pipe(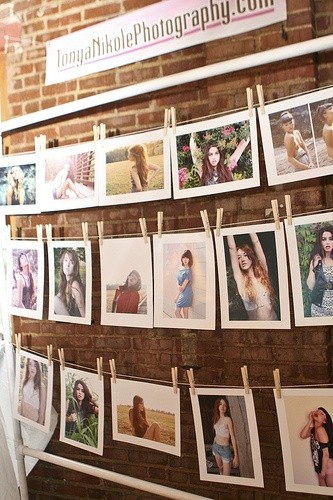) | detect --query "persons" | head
[16,226,333,317]
[5,103,333,206]
[20,358,333,487]
